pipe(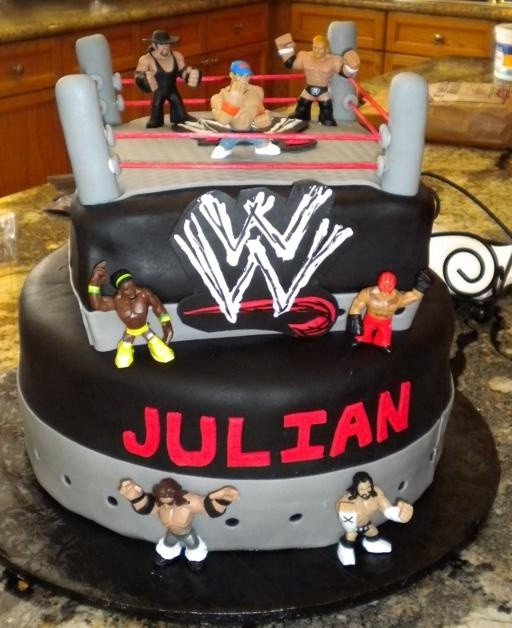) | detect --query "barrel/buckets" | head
[494,24,512,80]
